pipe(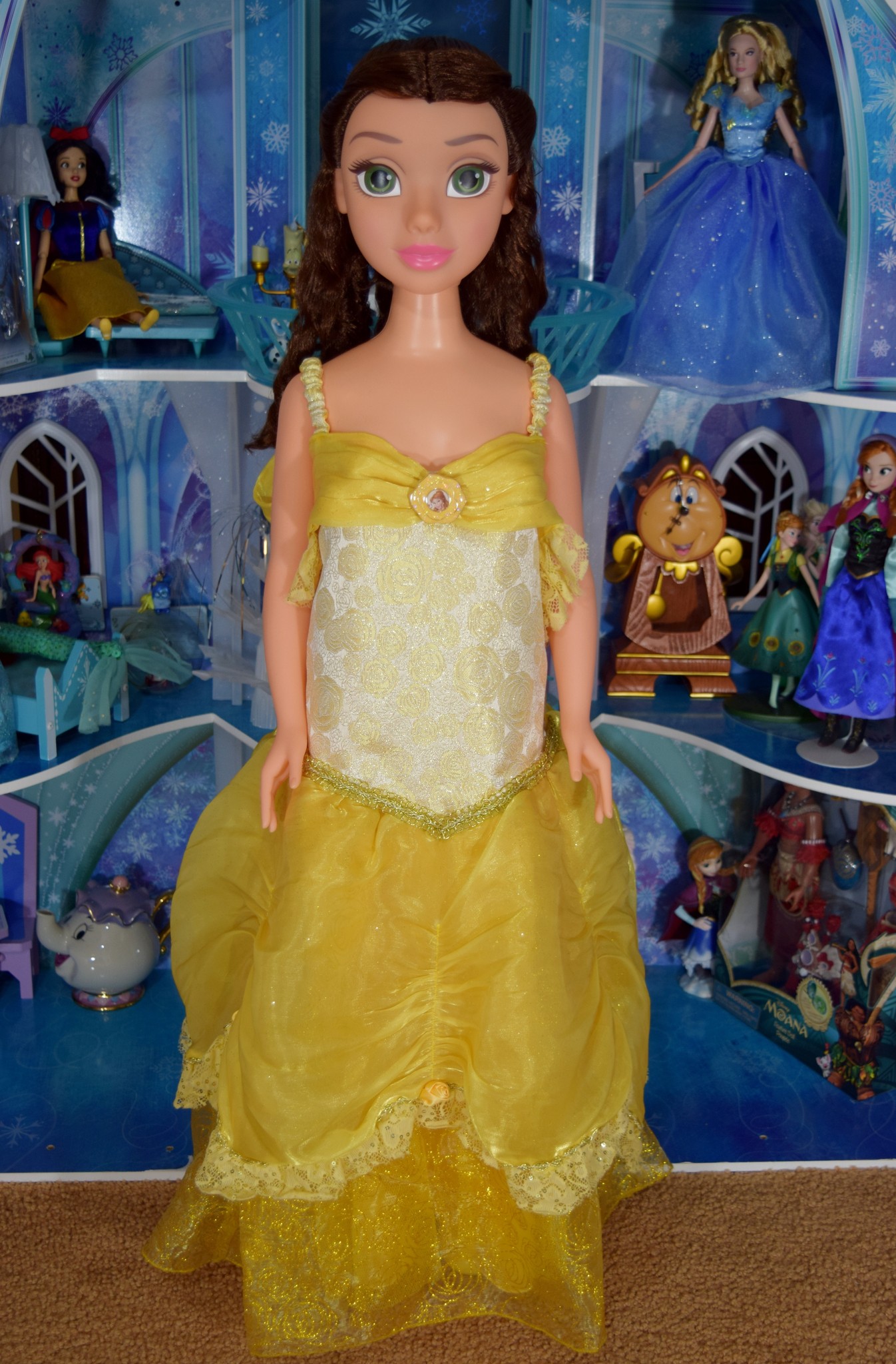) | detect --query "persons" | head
[3,427,895,1110]
[139,33,673,1364]
[31,123,159,348]
[585,14,849,400]
